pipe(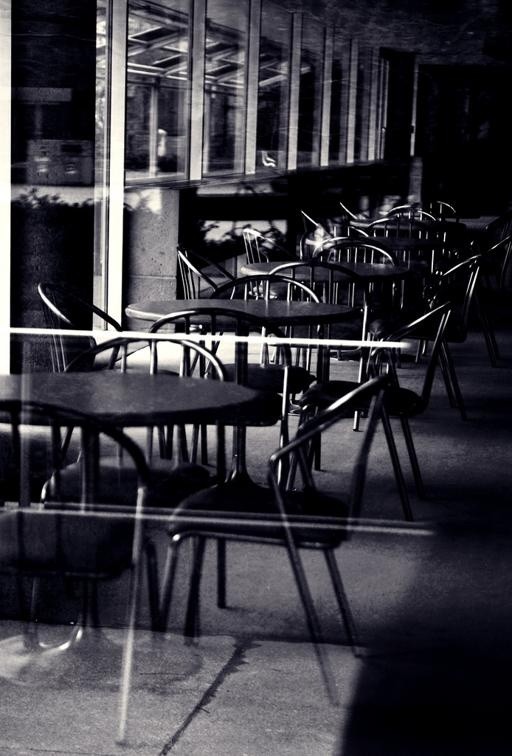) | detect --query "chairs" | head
[0,197,511,746]
